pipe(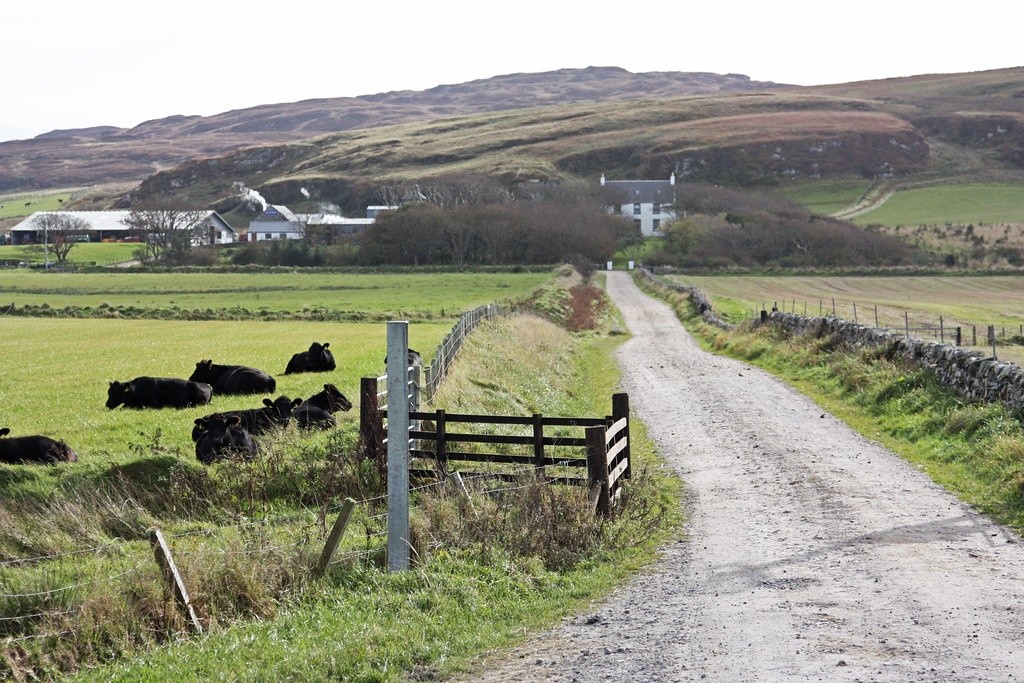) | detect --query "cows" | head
[0,428,79,467]
[383,348,425,375]
[278,342,336,376]
[189,359,276,396]
[106,376,213,411]
[191,383,353,464]
